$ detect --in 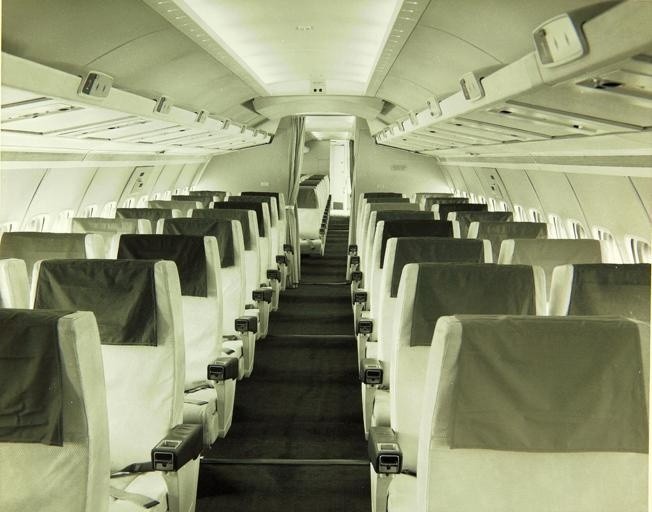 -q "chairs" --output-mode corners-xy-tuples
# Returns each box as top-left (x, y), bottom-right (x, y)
(187, 208), (272, 339)
(29, 257), (204, 511)
(358, 237), (494, 440)
(0, 258), (30, 309)
(351, 210), (440, 305)
(208, 201), (282, 311)
(1, 231), (106, 285)
(117, 233), (240, 445)
(2, 307), (111, 511)
(162, 217), (258, 378)
(366, 264), (546, 510)
(228, 195), (286, 292)
(543, 263), (650, 323)
(70, 173), (603, 265)
(416, 315), (651, 510)
(355, 219), (460, 351)
